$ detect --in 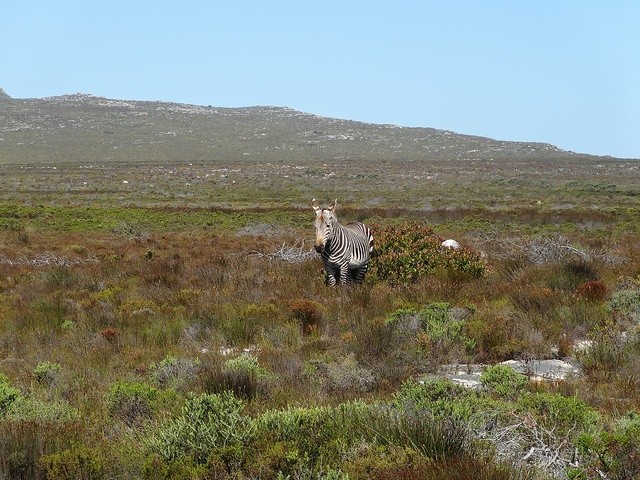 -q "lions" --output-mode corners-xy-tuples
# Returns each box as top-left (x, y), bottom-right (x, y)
(311, 198), (374, 287)
